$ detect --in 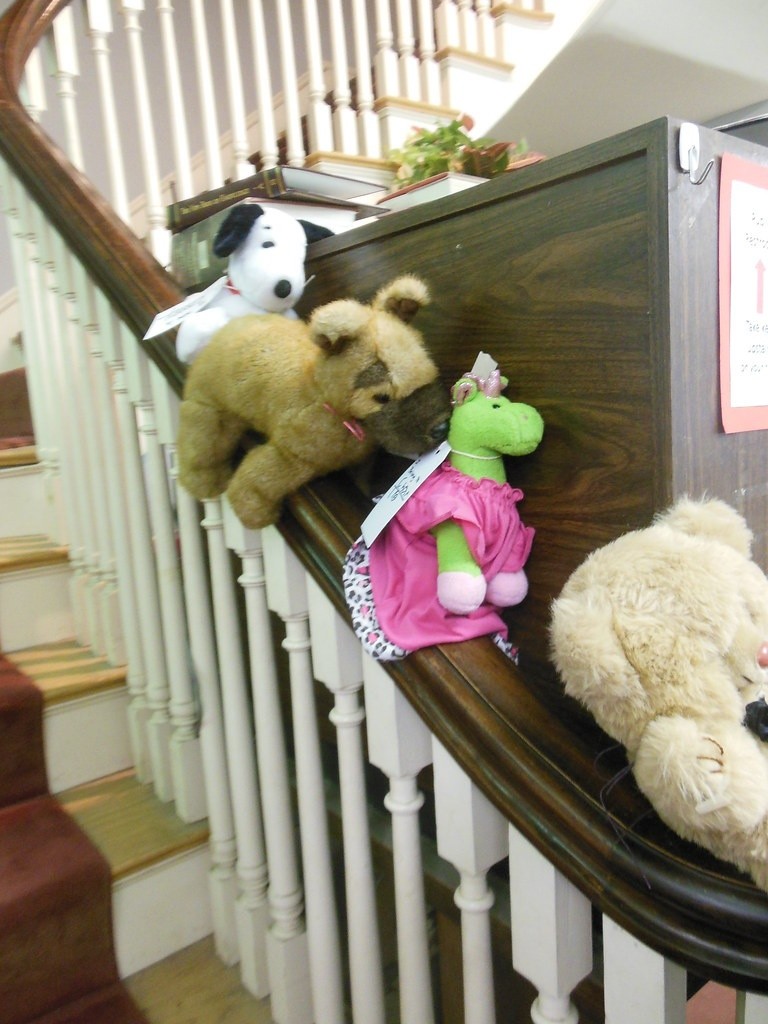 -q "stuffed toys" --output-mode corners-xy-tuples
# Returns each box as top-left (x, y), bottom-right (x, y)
(551, 498), (767, 892)
(343, 364), (543, 662)
(177, 269), (449, 530)
(174, 203), (336, 361)
